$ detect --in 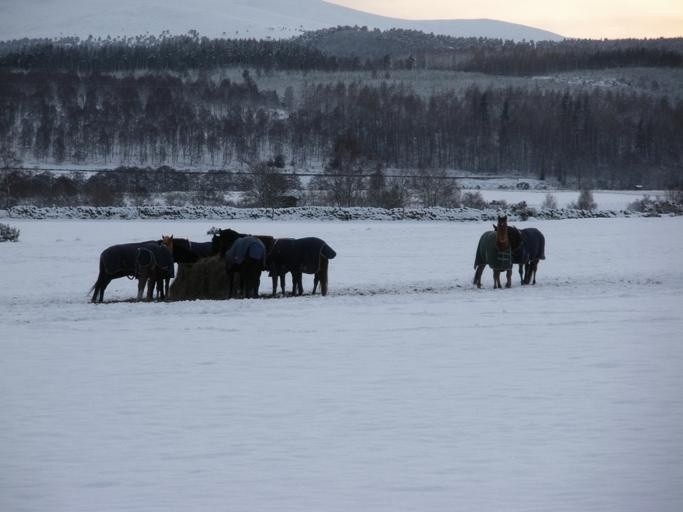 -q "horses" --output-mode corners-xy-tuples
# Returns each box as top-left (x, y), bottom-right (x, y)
(87, 228), (336, 302)
(472, 215), (512, 289)
(492, 223), (545, 288)
